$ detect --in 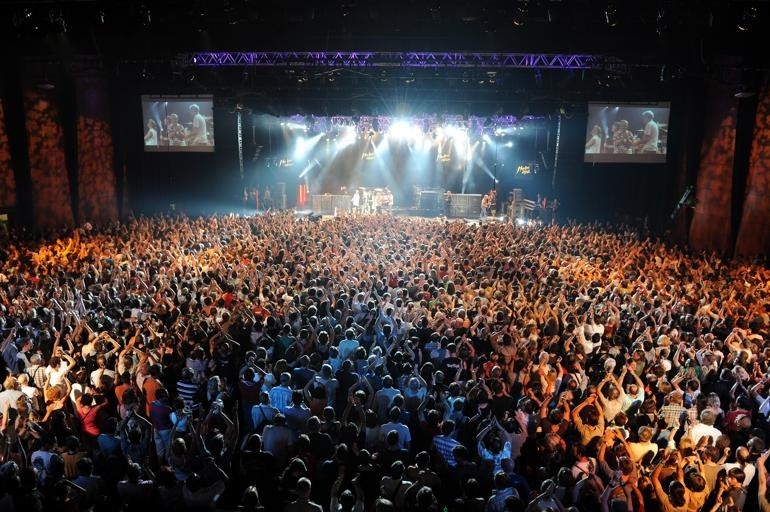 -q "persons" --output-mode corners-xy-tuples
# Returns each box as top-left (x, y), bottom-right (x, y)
(351, 187), (393, 215)
(0, 210), (770, 512)
(443, 190), (452, 215)
(145, 103), (210, 147)
(144, 104), (207, 147)
(586, 111), (658, 154)
(585, 110), (660, 156)
(480, 190), (498, 219)
